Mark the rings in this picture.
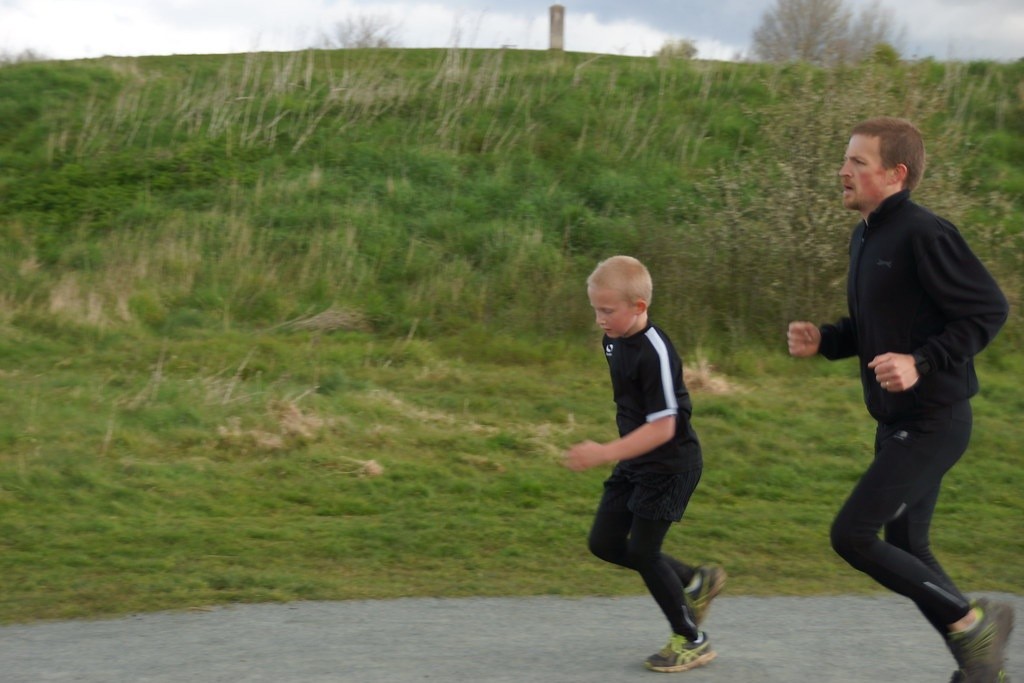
[886,381,888,385]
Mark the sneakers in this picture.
[684,560,727,626]
[644,631,717,673]
[942,598,1014,682]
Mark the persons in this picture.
[788,118,1016,682]
[558,255,727,672]
